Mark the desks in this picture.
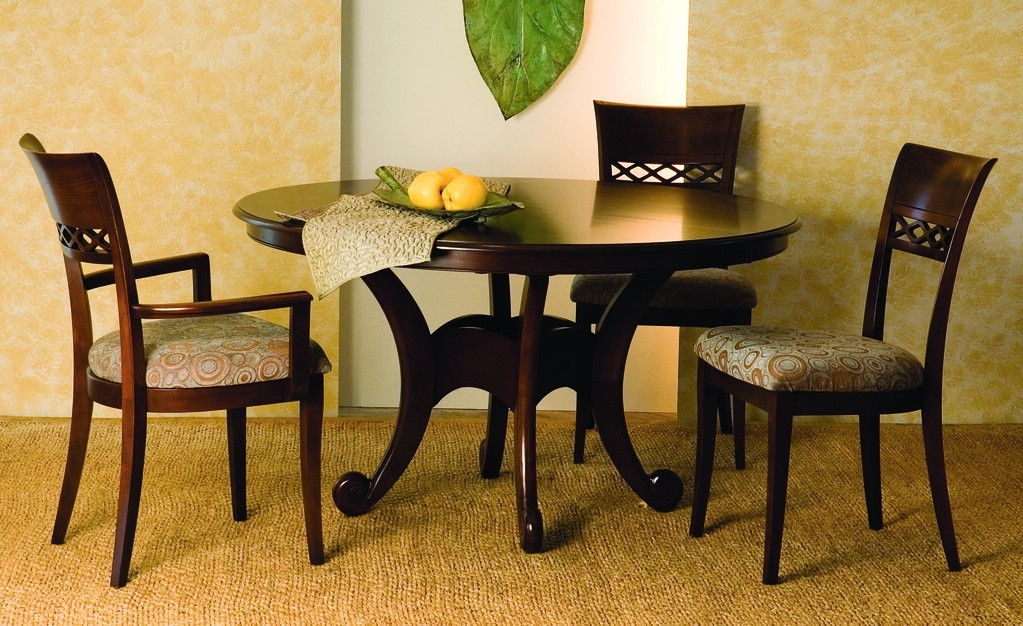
[229,177,806,558]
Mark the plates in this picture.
[373,165,525,217]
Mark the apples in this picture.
[408,167,488,210]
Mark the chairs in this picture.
[571,100,755,475]
[688,139,1002,585]
[19,129,338,594]
